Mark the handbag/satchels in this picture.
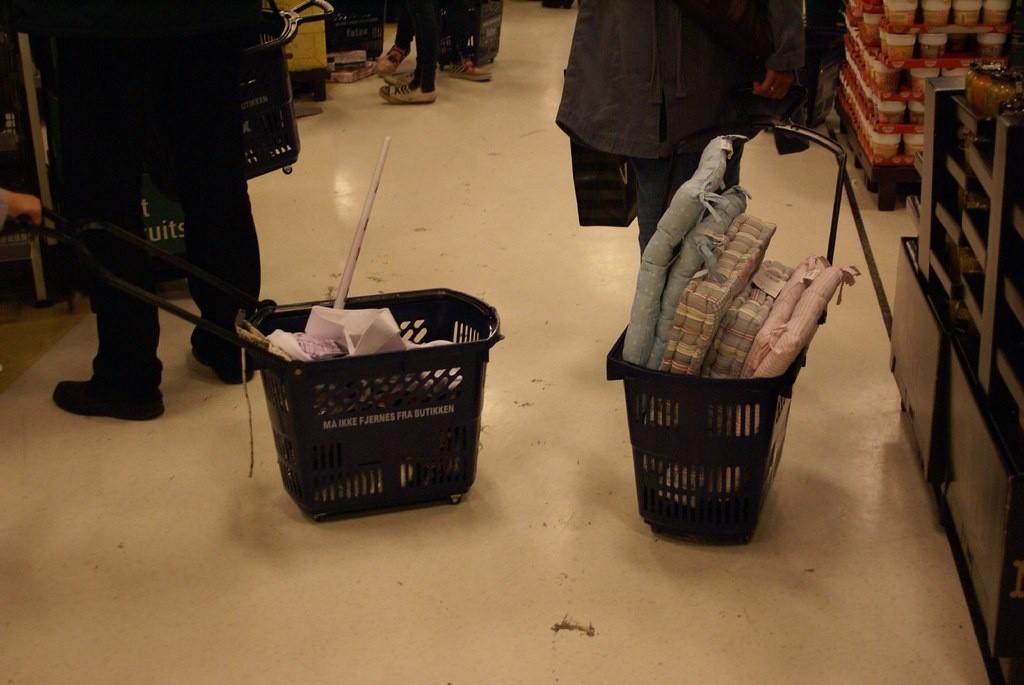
[569,136,636,227]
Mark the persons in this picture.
[0,189,44,231]
[2,1,262,423]
[555,0,814,285]
[373,0,492,104]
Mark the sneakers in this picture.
[448,60,492,81]
[379,85,436,104]
[385,74,414,86]
[376,44,411,75]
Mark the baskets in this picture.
[606,120,847,546]
[27,202,502,521]
[241,1,333,181]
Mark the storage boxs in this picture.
[327,50,377,83]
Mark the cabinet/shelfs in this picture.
[888,74,1024,685]
[0,31,59,301]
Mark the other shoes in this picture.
[52,379,165,421]
[193,346,253,383]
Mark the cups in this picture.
[838,0,1024,162]
